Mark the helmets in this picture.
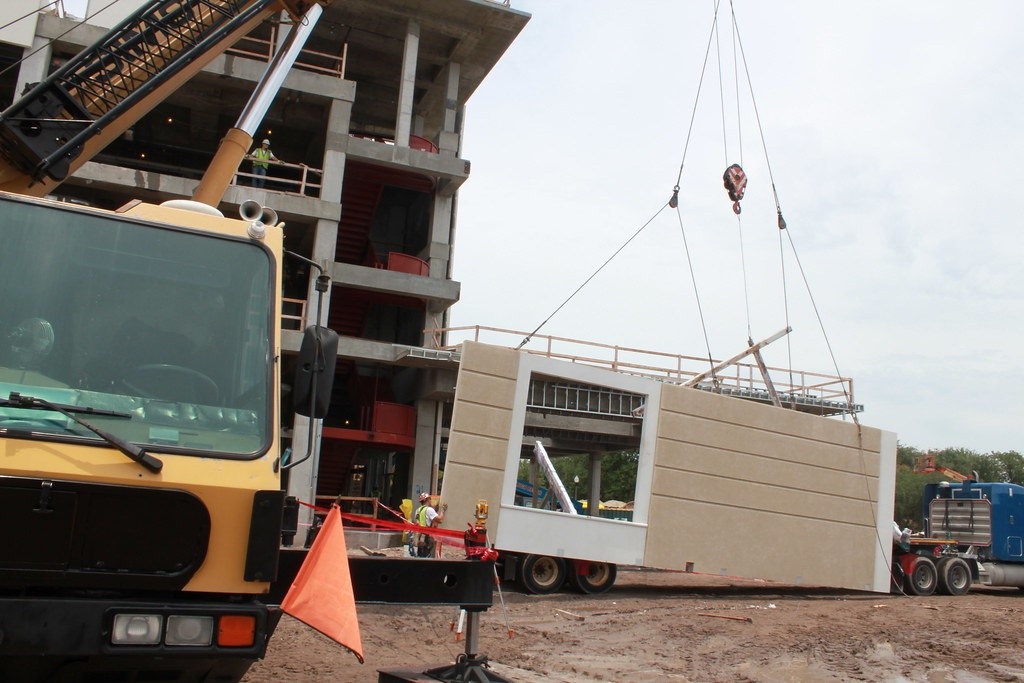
[418,492,431,501]
[263,139,270,145]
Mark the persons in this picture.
[415,493,448,557]
[248,139,284,188]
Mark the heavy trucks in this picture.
[493,471,1024,598]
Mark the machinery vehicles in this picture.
[0,0,514,683]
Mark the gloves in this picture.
[278,160,284,164]
[441,503,448,513]
